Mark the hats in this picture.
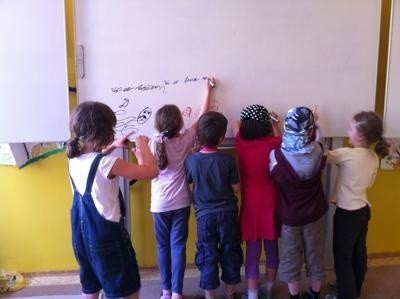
[240,103,270,125]
[283,106,315,136]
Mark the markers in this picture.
[270,114,280,123]
[208,79,215,88]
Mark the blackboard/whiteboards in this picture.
[73,0,382,143]
[0,0,71,144]
[381,0,400,138]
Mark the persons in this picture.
[233,104,281,299]
[268,104,329,299]
[147,78,215,299]
[65,101,158,299]
[324,110,389,299]
[185,111,244,299]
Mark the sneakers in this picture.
[241,278,272,298]
[302,286,320,299]
[325,279,354,299]
